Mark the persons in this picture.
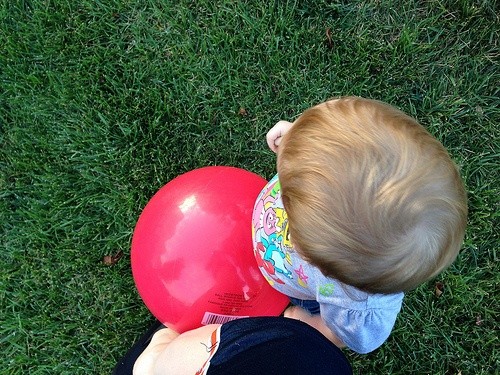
[116,310,354,375]
[250,93,469,355]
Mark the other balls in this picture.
[130,167,290,334]
